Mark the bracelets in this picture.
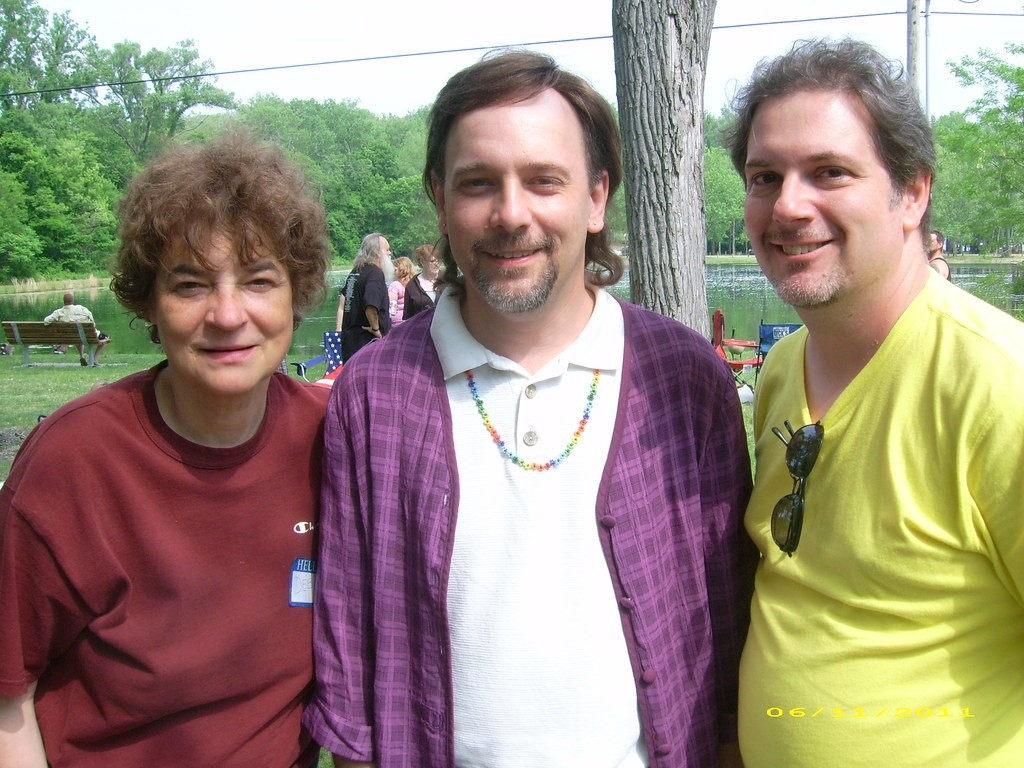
[371,327,379,332]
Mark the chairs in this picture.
[710,308,804,389]
[292,331,343,384]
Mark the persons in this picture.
[0,120,336,768]
[402,243,446,321]
[337,233,395,366]
[44,294,109,366]
[302,50,760,768]
[386,256,416,327]
[927,231,952,283]
[724,36,1024,768]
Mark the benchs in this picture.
[1,321,112,367]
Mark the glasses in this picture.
[771,419,825,556]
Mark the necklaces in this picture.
[464,365,600,470]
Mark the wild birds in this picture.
[0,342,16,354]
[52,344,69,355]
